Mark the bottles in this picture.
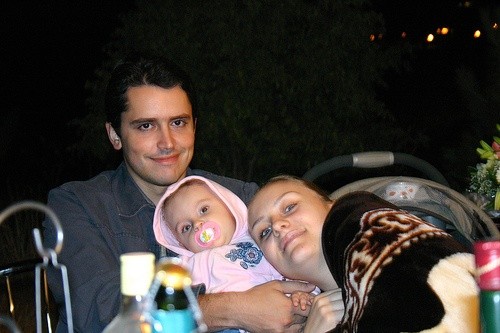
[149,263,198,333]
[474,241,500,333]
[102,252,155,333]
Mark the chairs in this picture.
[300,151,500,254]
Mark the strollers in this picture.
[302,151,499,245]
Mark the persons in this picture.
[153,176,322,333]
[44,56,316,332]
[248,176,481,333]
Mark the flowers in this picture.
[467,124,500,212]
